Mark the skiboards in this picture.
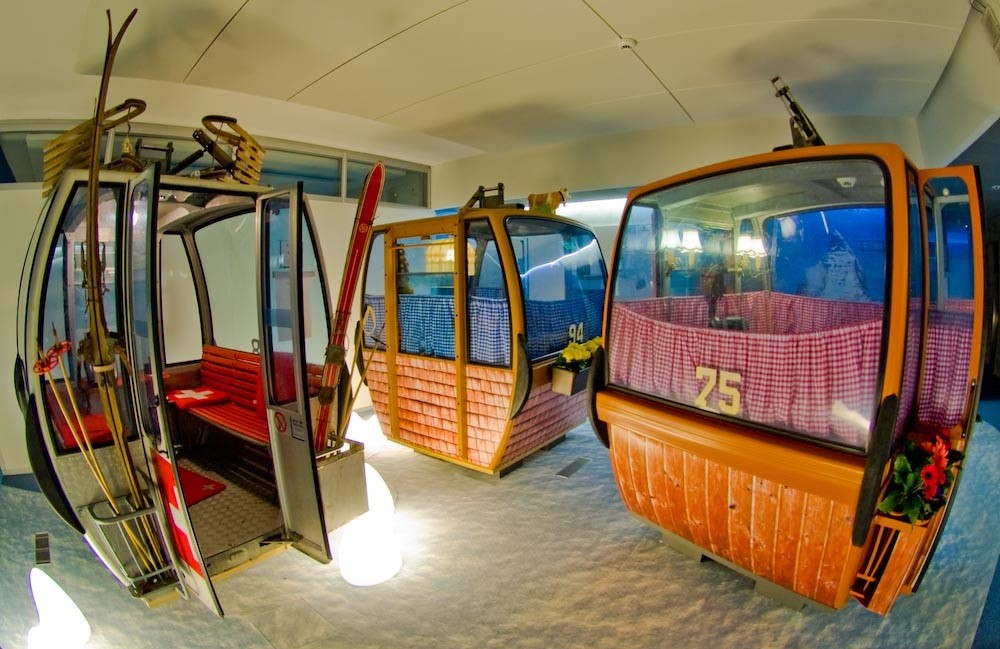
[85,6,180,580]
[311,159,387,461]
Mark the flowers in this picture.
[876,435,966,520]
[556,336,601,370]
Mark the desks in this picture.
[104,373,169,391]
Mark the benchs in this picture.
[172,345,325,447]
[46,393,132,452]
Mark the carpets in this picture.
[178,466,226,507]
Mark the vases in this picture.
[550,365,591,396]
[853,491,949,616]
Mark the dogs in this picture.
[527,186,571,215]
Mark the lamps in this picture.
[727,236,770,277]
[662,228,704,277]
